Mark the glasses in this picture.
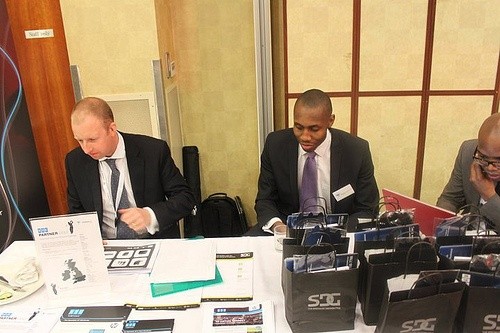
[473,145,500,169]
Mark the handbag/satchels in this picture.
[281,195,500,333]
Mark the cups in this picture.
[274,226,288,252]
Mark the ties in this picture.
[104,159,135,239]
[301,151,318,212]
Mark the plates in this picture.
[0,257,46,306]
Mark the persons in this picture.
[436,111,500,238]
[242,89,379,237]
[65,97,195,245]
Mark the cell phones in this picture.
[479,165,487,176]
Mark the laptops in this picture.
[382,188,458,237]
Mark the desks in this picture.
[0,229,375,333]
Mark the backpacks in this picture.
[201,193,240,238]
